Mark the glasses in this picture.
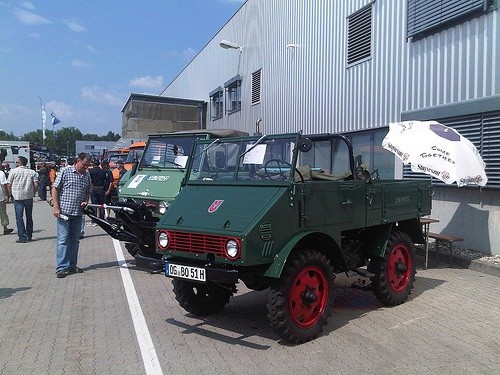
[80,160,90,166]
[16,162,20,164]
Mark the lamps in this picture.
[219,39,242,52]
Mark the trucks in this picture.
[81,118,263,259]
[59,139,168,201]
[154,129,432,344]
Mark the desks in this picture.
[417,218,440,269]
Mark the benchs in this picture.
[423,231,464,268]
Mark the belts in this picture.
[92,185,104,188]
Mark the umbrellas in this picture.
[381,119,488,269]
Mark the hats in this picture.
[171,144,179,150]
[115,160,124,165]
[101,160,109,164]
[91,159,100,164]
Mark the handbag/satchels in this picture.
[47,198,53,206]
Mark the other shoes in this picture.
[65,267,83,273]
[57,271,65,278]
[85,222,98,226]
[4,229,14,234]
[16,240,26,243]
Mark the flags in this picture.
[50,112,61,125]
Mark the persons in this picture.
[51,152,91,277]
[0,159,14,235]
[6,156,39,243]
[2,155,127,227]
